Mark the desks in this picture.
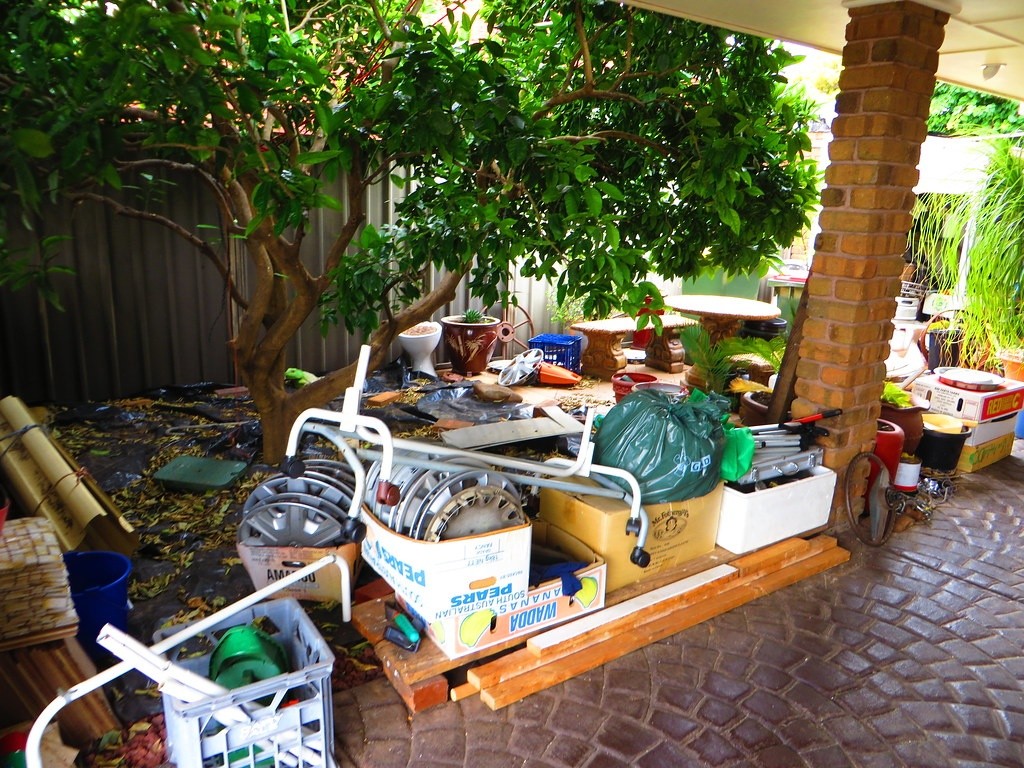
[664,295,781,397]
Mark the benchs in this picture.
[724,351,783,427]
[570,315,698,382]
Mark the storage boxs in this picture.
[612,373,658,405]
[60,551,131,657]
[631,380,689,402]
[921,291,954,319]
[963,411,1019,446]
[396,517,607,660]
[359,501,531,618]
[928,330,962,373]
[528,333,582,375]
[235,540,359,603]
[915,421,973,470]
[715,465,838,556]
[956,431,1015,473]
[863,419,905,513]
[533,477,723,593]
[912,369,1024,424]
[1014,408,1024,440]
[149,597,337,768]
[892,295,918,320]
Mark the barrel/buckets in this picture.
[62,552,132,661]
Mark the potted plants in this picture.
[441,309,501,377]
[880,380,930,455]
[995,338,1024,382]
[739,388,772,426]
[893,452,921,492]
[397,319,443,377]
